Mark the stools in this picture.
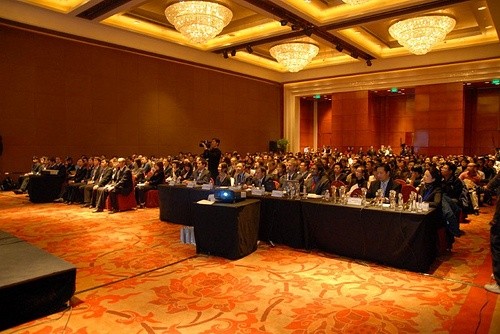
[67,182,161,210]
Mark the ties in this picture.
[382,184,386,197]
[288,175,292,180]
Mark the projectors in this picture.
[214,188,246,199]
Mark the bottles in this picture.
[325,190,330,201]
[251,184,255,194]
[236,183,242,192]
[209,180,212,189]
[177,178,180,184]
[260,185,265,195]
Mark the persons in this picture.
[202,138,222,172]
[482,170,500,293]
[14,143,500,251]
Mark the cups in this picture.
[423,203,429,211]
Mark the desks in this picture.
[157,182,442,275]
[193,199,260,260]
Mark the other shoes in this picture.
[109,209,118,214]
[84,203,96,209]
[475,210,479,215]
[92,208,103,213]
[14,189,23,194]
[484,283,500,294]
[64,200,73,205]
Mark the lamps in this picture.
[388,13,456,55]
[268,42,320,72]
[163,0,234,46]
